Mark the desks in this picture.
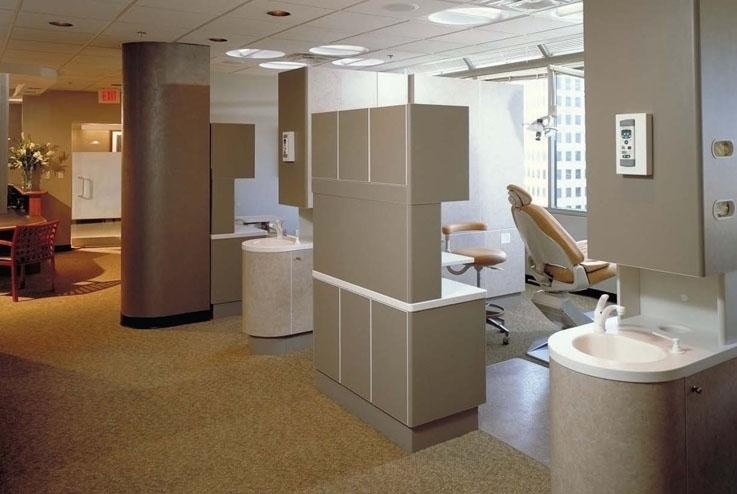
[0,213,47,274]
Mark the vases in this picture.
[19,168,33,189]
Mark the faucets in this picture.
[268,219,283,240]
[594,293,626,333]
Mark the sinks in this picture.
[573,331,668,364]
[250,237,293,248]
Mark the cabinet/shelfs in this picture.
[682,359,737,493]
[210,122,284,305]
[310,106,489,454]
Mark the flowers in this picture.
[6,132,57,168]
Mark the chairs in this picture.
[0,220,59,301]
[507,183,617,363]
[442,221,511,345]
